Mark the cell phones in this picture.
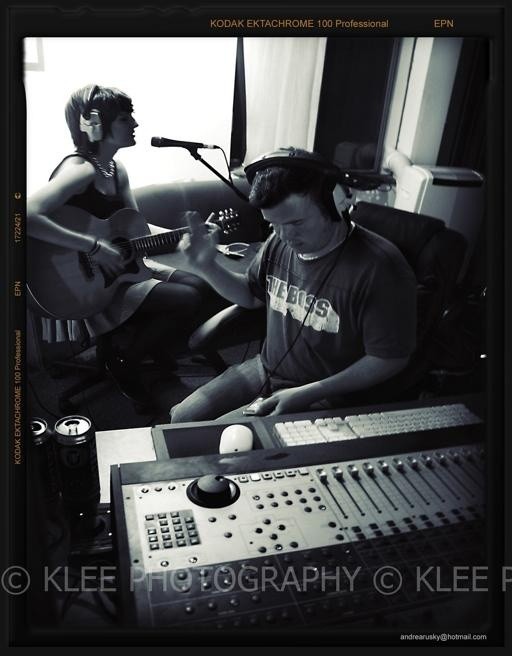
[242,393,272,415]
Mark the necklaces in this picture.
[91,153,116,178]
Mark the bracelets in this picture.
[84,236,103,256]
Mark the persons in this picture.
[27,87,205,398]
[171,153,421,428]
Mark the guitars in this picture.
[19,204,241,321]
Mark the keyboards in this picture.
[271,401,487,450]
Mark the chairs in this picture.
[350,199,450,406]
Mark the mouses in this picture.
[215,423,253,454]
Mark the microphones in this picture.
[149,134,216,159]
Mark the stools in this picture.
[27,286,164,415]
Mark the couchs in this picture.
[130,173,267,345]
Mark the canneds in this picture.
[53,415,100,508]
[28,417,58,502]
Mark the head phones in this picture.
[74,84,106,165]
[240,148,360,221]
